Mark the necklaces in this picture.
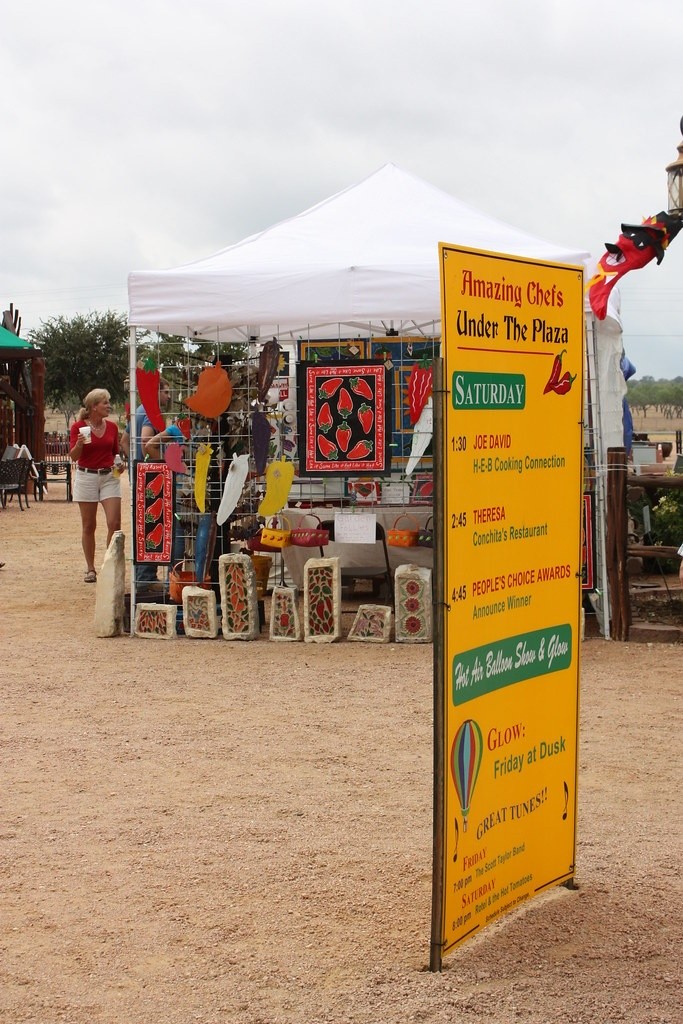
[89,420,105,430]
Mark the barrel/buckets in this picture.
[249,555,272,596]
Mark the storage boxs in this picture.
[122,592,169,633]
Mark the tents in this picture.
[126,163,628,638]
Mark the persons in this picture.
[70,389,125,583]
[120,377,171,592]
[145,421,200,573]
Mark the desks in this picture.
[36,462,74,501]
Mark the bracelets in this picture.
[76,443,83,447]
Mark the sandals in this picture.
[84,570,97,582]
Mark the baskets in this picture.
[290,514,329,547]
[387,514,419,547]
[169,561,211,603]
[418,515,433,547]
[260,515,291,548]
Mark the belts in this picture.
[79,467,114,475]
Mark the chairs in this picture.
[316,519,393,614]
[0,458,26,511]
[6,458,34,508]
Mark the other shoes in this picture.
[0,562,5,568]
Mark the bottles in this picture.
[113,453,122,478]
[350,483,357,506]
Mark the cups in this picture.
[79,426,91,444]
